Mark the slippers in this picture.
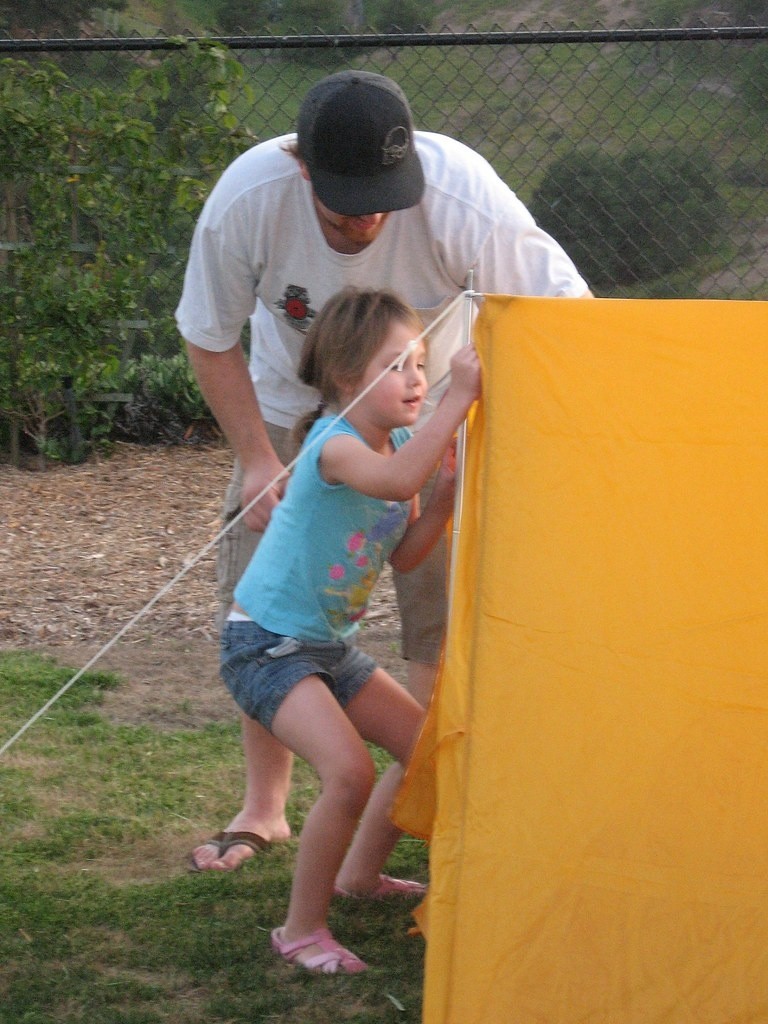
[271,927,369,975]
[185,831,271,874]
[333,874,426,901]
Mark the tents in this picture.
[386,299,768,1024]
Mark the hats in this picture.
[297,70,426,212]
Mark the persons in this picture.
[175,70,595,873]
[220,285,482,975]
